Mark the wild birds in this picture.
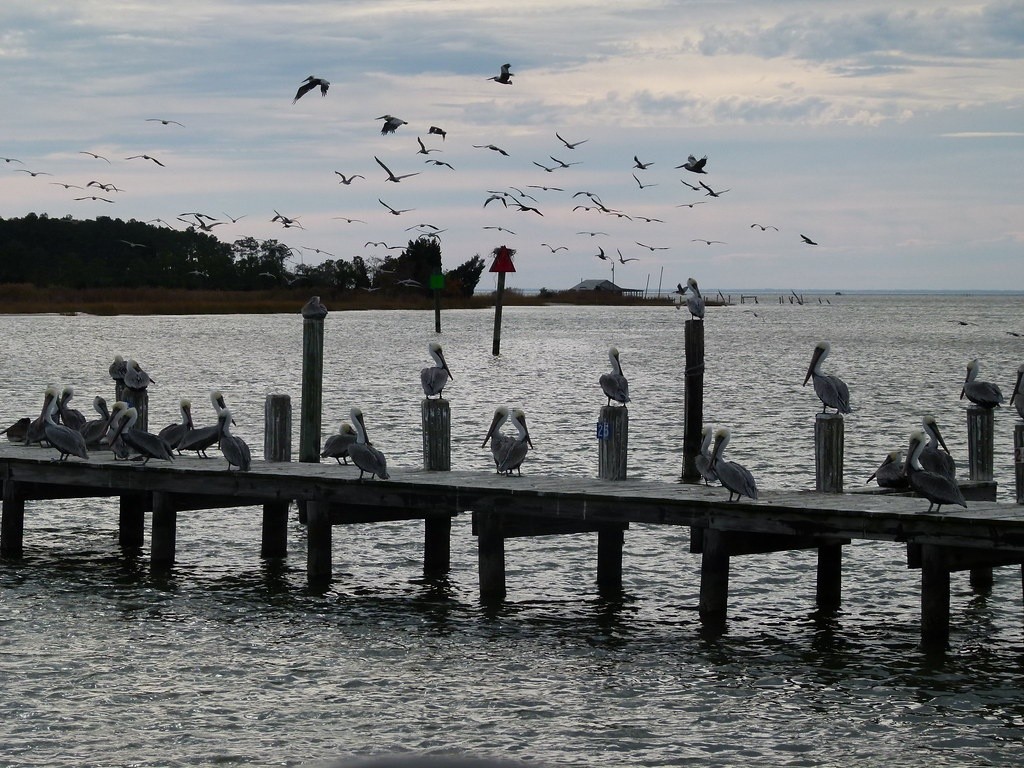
[686,277,705,319]
[348,406,390,482]
[1,357,236,466]
[918,414,956,480]
[673,283,689,295]
[901,430,968,513]
[706,428,758,503]
[294,62,821,275]
[318,424,358,465]
[867,450,912,488]
[420,342,454,400]
[599,347,632,407]
[949,319,977,327]
[481,406,534,478]
[803,340,853,415]
[1009,364,1024,420]
[0,118,306,279]
[960,358,1004,407]
[218,409,251,472]
[695,426,719,487]
